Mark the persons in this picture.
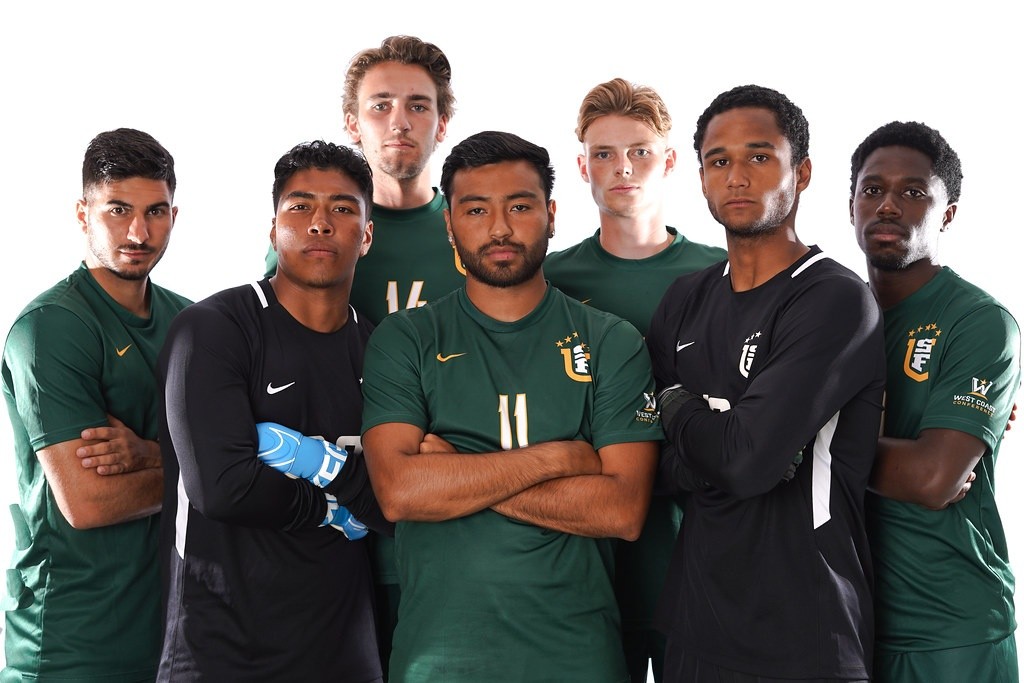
[156,139,383,683]
[645,85,888,683]
[0,127,195,683]
[360,131,668,683]
[542,78,728,683]
[263,36,466,683]
[848,122,1023,683]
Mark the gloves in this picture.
[781,444,807,482]
[651,368,683,406]
[256,422,348,488]
[318,492,369,540]
